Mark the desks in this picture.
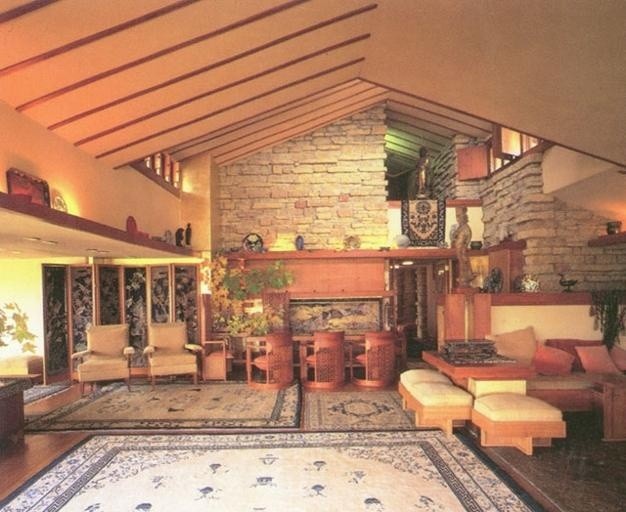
[420,349,537,393]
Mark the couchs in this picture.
[522,339,626,415]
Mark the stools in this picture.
[0,354,42,390]
[397,369,568,457]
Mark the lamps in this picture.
[386,143,432,201]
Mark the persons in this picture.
[416,147,429,194]
[451,205,473,288]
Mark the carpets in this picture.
[0,425,550,512]
[17,380,303,435]
[298,376,444,433]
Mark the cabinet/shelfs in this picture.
[0,381,27,454]
[589,378,626,445]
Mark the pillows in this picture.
[485,325,626,378]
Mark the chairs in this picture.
[201,322,400,391]
[143,322,203,390]
[70,322,135,398]
